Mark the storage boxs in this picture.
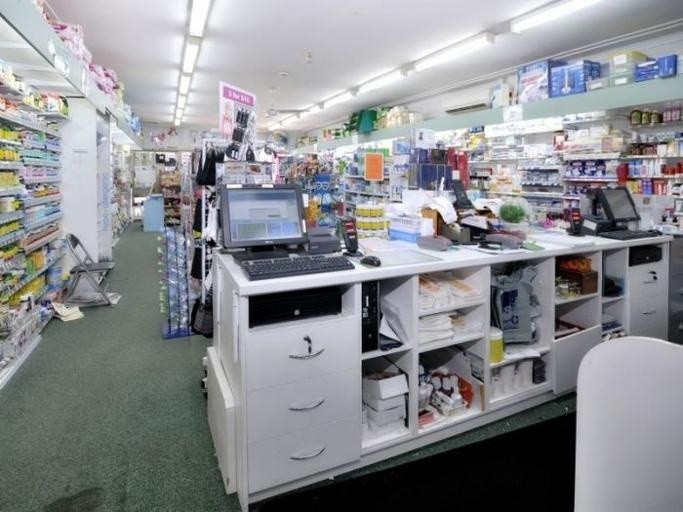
[585,51,683,92]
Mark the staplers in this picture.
[479,232,521,250]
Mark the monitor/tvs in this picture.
[222,183,309,262]
[598,186,642,231]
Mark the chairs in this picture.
[63,233,115,308]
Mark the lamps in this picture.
[267,65,409,133]
[413,32,495,72]
[509,0,600,33]
[174,0,214,127]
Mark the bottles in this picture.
[627,102,683,194]
[489,325,505,364]
[448,386,463,406]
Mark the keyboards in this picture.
[239,255,355,280]
[602,228,653,241]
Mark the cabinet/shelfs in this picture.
[207,235,675,512]
[1,76,68,388]
[344,98,683,237]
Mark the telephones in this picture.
[453,180,474,208]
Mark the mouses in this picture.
[360,255,382,267]
[648,228,662,235]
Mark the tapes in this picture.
[448,223,465,231]
